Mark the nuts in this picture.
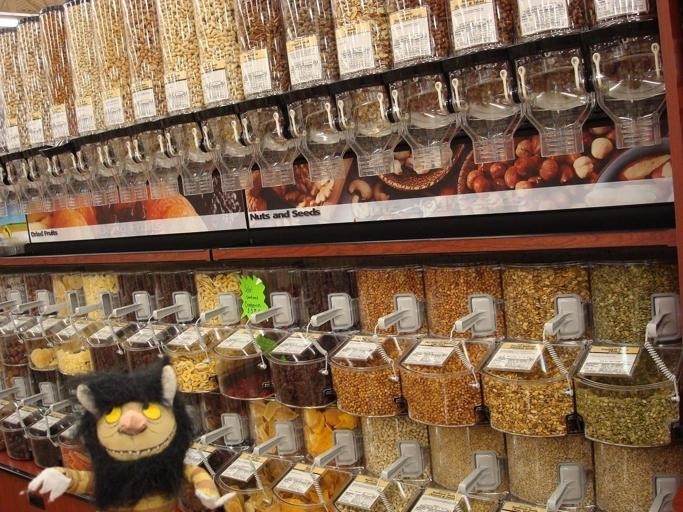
[30,126,679,511]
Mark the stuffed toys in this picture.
[28,353,237,512]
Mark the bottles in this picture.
[0,0,667,219]
